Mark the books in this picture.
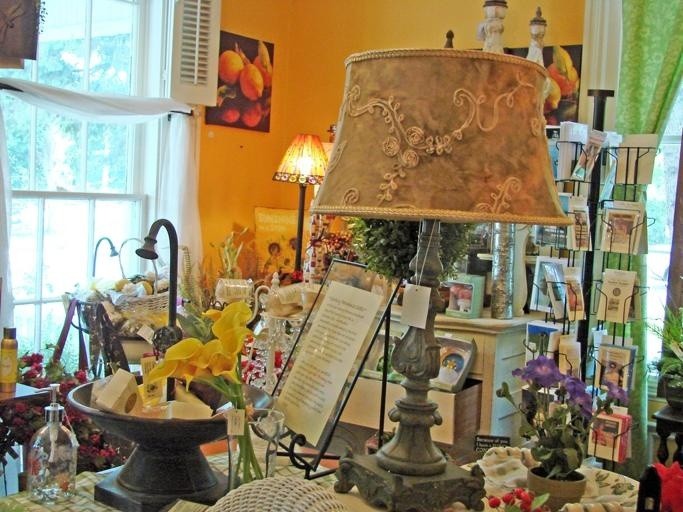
[525,321,561,367]
[593,414,631,461]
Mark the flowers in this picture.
[493,331,629,480]
[145,300,263,493]
[487,486,549,512]
[0,343,118,476]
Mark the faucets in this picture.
[136,217,179,325]
[91,238,117,278]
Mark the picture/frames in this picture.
[528,256,570,314]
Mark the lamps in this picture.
[311,47,574,512]
[272,134,327,281]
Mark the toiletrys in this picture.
[29,384,80,505]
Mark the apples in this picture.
[451,286,472,299]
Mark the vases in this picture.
[523,466,585,512]
[222,407,285,498]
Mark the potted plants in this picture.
[636,300,683,414]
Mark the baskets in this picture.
[102,237,169,314]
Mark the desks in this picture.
[0,382,509,512]
[338,304,550,445]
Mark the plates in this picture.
[575,465,639,512]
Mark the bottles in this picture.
[25,405,76,504]
[0,326,18,396]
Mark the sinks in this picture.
[68,375,273,493]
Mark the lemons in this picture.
[207,50,273,127]
[116,278,130,291]
[138,281,153,295]
[542,60,578,127]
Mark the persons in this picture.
[445,281,465,311]
[603,361,619,387]
[281,237,296,274]
[263,241,281,274]
[455,288,472,312]
[587,142,599,158]
